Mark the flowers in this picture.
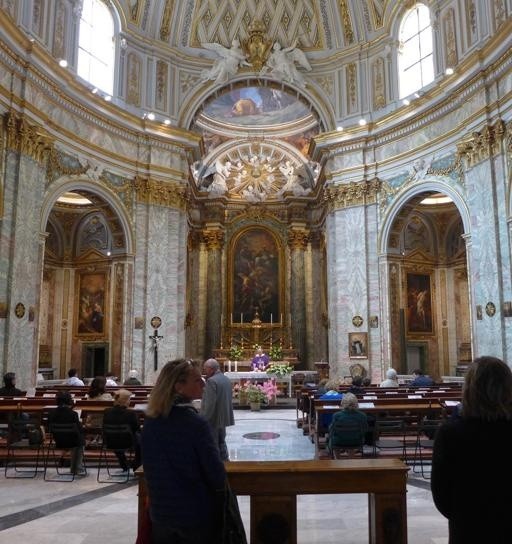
[232,383,280,401]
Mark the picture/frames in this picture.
[349,333,368,359]
[73,269,110,340]
[401,266,435,340]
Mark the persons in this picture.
[85,376,112,401]
[139,359,248,544]
[250,348,269,371]
[429,356,512,544]
[0,372,21,394]
[123,370,142,385]
[200,358,236,462]
[101,389,142,473]
[63,367,85,386]
[48,391,86,475]
[105,372,118,386]
[324,392,369,453]
[318,367,434,427]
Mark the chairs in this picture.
[329,419,440,479]
[7,417,142,484]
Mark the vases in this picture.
[249,401,261,412]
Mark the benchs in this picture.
[1,383,155,457]
[294,383,462,460]
